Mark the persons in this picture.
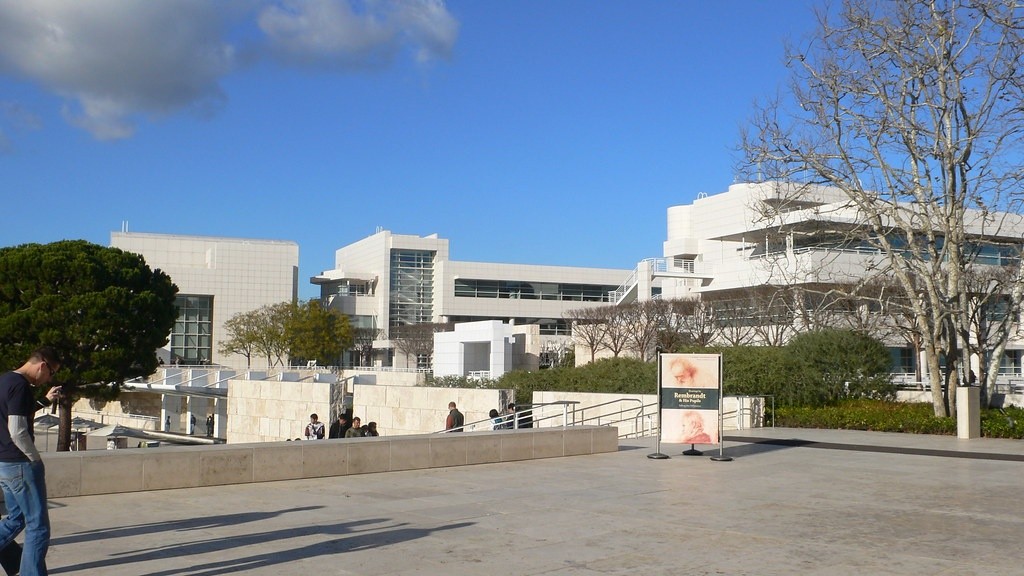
[443,402,466,432]
[164,415,214,438]
[285,415,380,441]
[670,358,698,388]
[489,404,522,430]
[136,442,162,447]
[0,348,65,575]
[159,357,164,364]
[677,411,712,444]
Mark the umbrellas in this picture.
[82,422,154,450]
[34,412,112,452]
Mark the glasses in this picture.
[42,358,55,376]
